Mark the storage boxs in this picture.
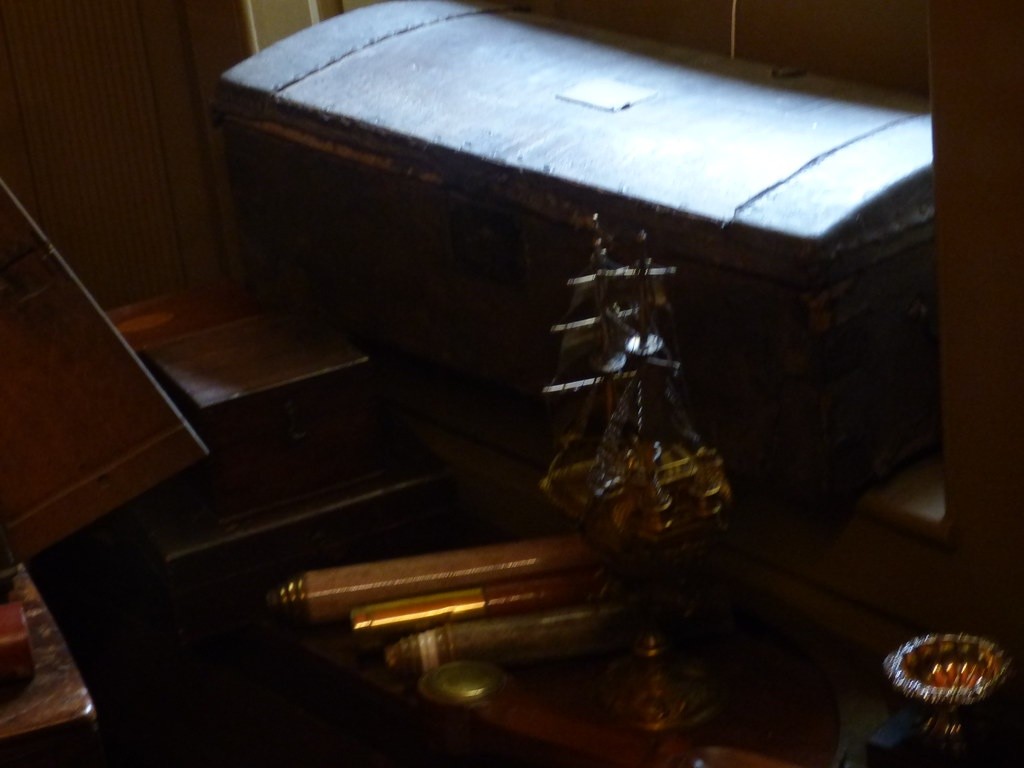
[121,0,934,653]
